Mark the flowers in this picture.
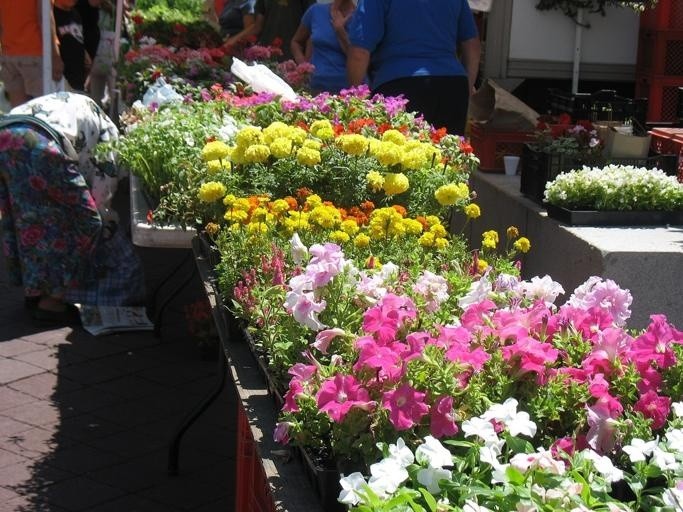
[196,187,682,512]
[96,0,483,226]
[516,110,682,225]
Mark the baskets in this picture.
[467,88,682,205]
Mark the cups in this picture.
[502,156,523,177]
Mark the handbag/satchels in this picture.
[64,220,146,311]
[89,30,116,79]
[469,78,540,133]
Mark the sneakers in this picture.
[25,296,81,320]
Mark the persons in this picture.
[53,2,133,120]
[198,1,482,138]
[0,89,120,328]
[0,0,64,108]
